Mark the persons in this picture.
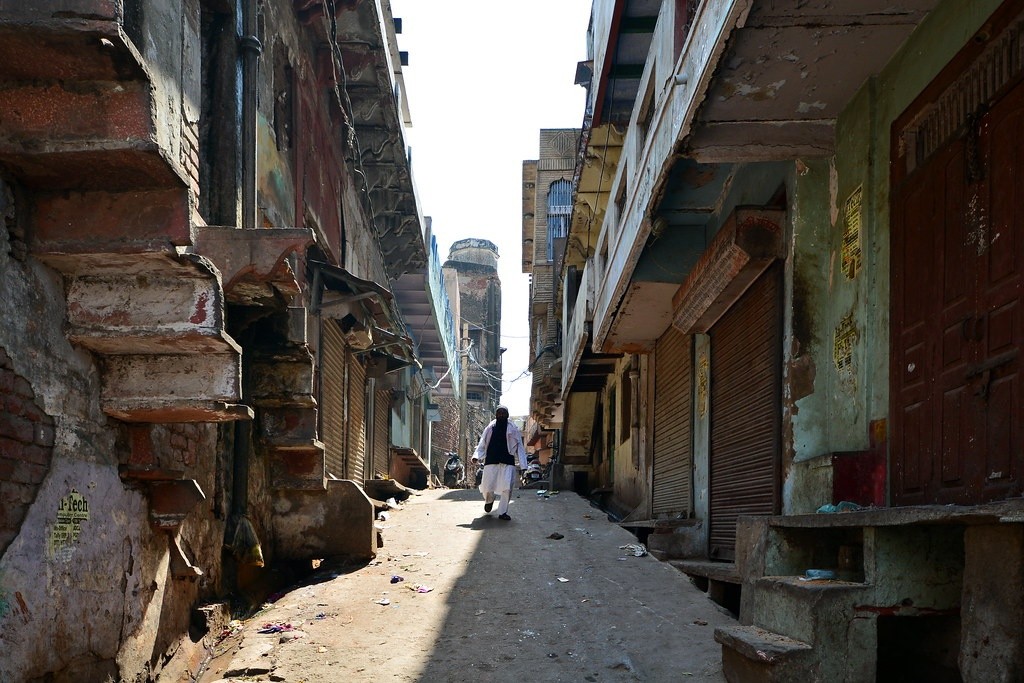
[471,405,527,520]
[444,454,461,488]
[474,463,484,488]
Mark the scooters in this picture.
[474,459,484,487]
[520,452,542,488]
[443,452,464,488]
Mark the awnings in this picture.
[309,259,422,373]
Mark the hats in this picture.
[496,405,508,412]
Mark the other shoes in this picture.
[499,513,511,520]
[485,502,493,512]
